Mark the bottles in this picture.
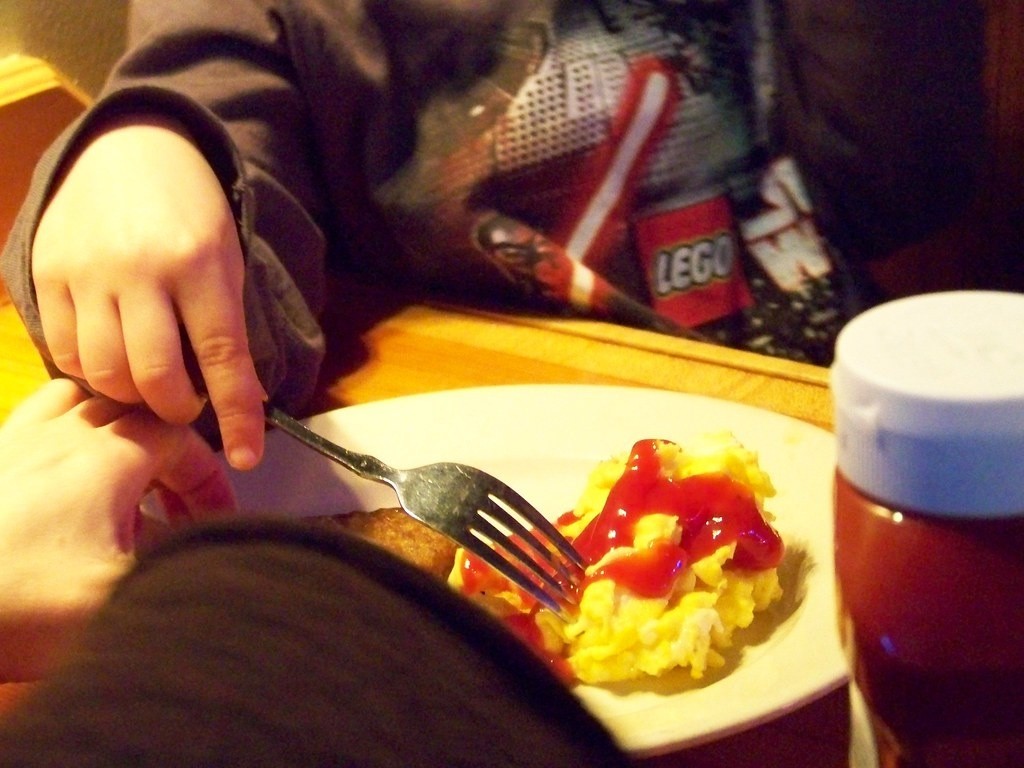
[830,289,1022,768]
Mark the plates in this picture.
[133,380,852,758]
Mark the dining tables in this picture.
[5,270,853,768]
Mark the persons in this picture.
[3,379,638,768]
[2,2,982,474]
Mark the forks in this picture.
[177,344,591,625]
